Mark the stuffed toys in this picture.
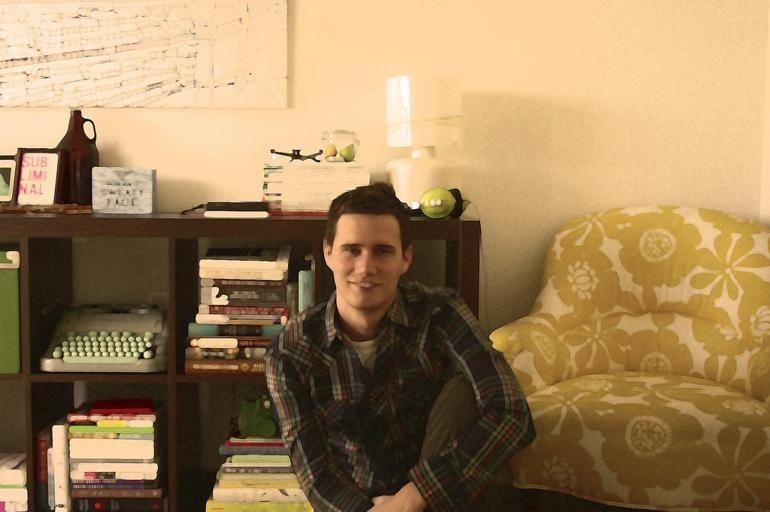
[237,394,281,438]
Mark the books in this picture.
[182,244,315,374]
[0,396,166,512]
[203,201,272,219]
[205,435,315,511]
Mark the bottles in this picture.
[386,146,455,205]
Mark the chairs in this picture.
[480,201,770,511]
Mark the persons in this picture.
[262,181,536,511]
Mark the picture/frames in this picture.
[9,146,65,208]
[0,153,20,208]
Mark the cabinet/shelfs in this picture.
[0,196,482,512]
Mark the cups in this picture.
[322,128,359,162]
